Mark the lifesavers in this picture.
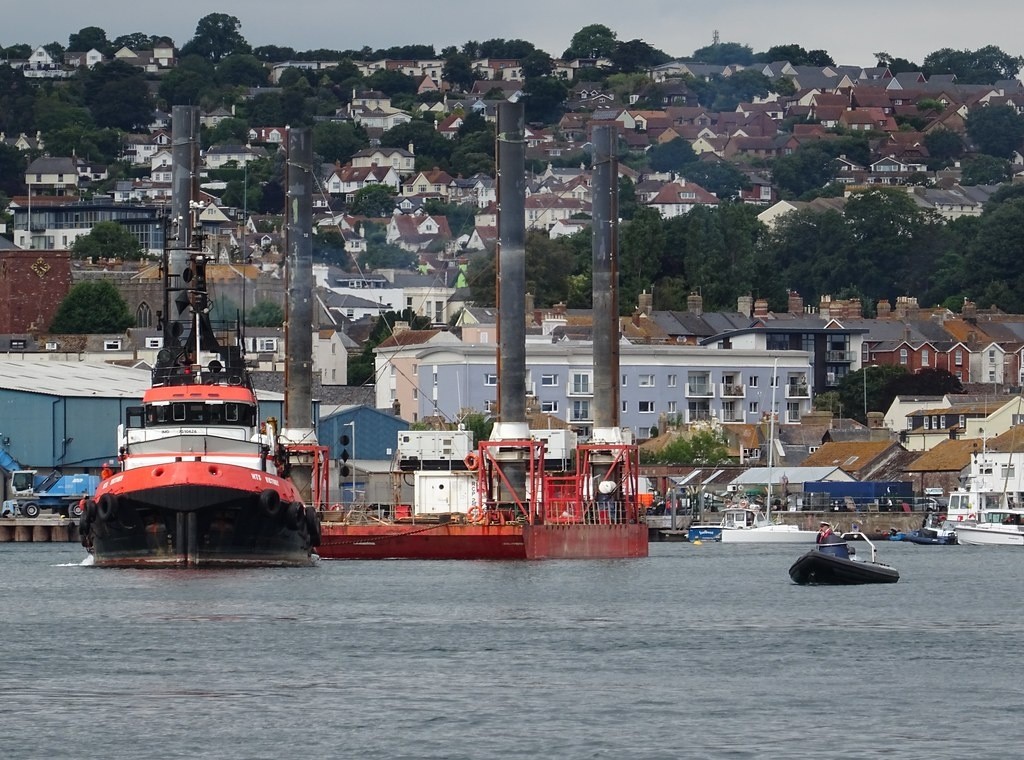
[469,506,485,522]
[464,453,480,469]
[330,505,344,511]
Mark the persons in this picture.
[816,522,835,544]
[101,463,113,480]
[676,498,679,515]
[666,499,671,516]
[887,498,893,512]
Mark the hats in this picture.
[819,521,830,527]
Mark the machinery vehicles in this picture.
[0,445,103,517]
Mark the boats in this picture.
[73,207,1024,563]
[788,530,899,584]
